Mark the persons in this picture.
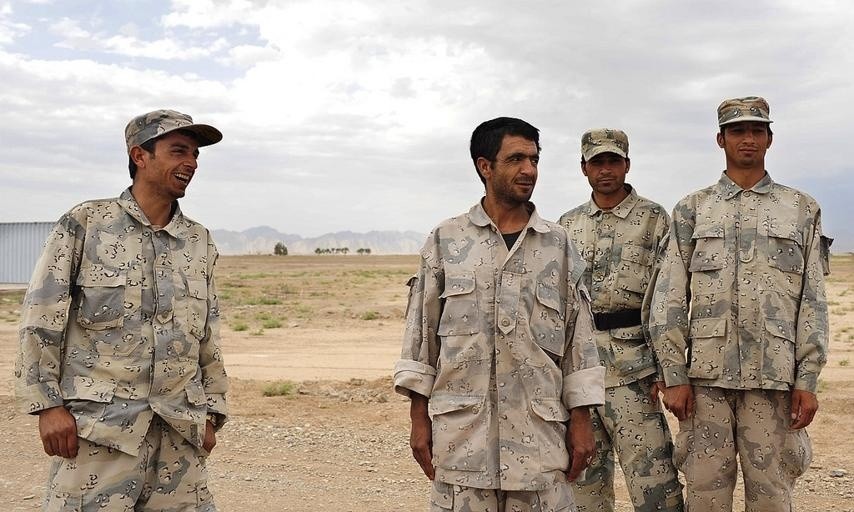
[393,118,607,512]
[669,96,834,511]
[13,109,231,512]
[554,129,685,512]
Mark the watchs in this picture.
[207,414,218,427]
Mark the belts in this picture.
[591,308,643,331]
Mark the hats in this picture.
[716,96,772,126]
[123,109,223,154]
[580,129,629,162]
